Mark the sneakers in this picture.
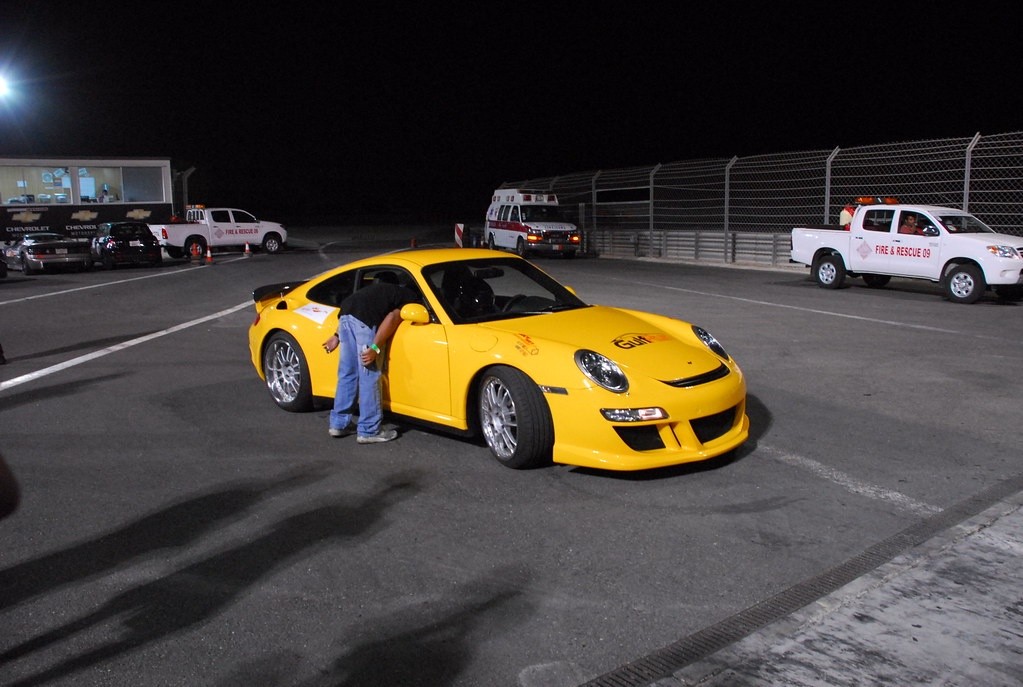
[357,427,398,443]
[329,419,358,436]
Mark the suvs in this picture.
[484,188,582,260]
[91,222,162,270]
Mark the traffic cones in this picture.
[203,247,221,268]
[243,241,257,260]
[190,242,203,260]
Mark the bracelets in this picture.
[371,345,381,354]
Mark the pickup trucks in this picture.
[146,205,288,258]
[788,204,1023,306]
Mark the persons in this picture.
[899,212,925,235]
[322,281,419,444]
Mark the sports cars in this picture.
[248,247,751,473]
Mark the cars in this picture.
[0,232,93,275]
